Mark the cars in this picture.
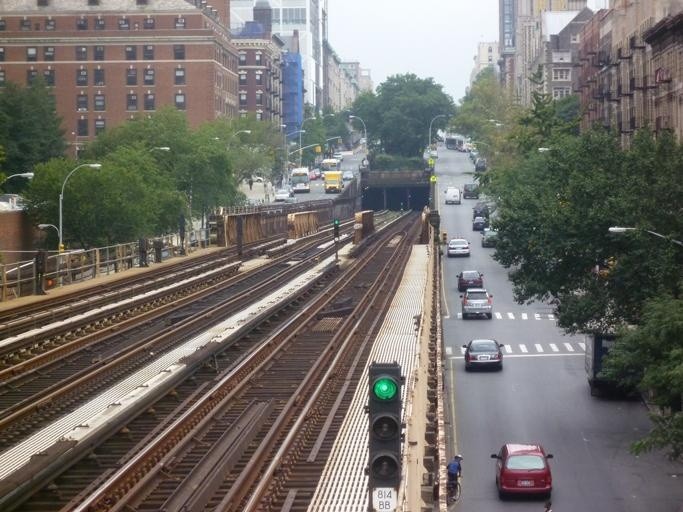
[439,126,505,372]
[274,152,353,203]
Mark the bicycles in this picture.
[446,473,461,505]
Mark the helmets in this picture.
[454,455,463,460]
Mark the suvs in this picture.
[490,442,554,500]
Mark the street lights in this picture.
[35,164,102,287]
[608,227,682,248]
[428,114,453,160]
[536,145,552,154]
[147,146,170,153]
[488,119,505,130]
[186,137,222,219]
[225,114,368,174]
[0,172,34,186]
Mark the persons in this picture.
[445,453,463,501]
[544,500,553,512]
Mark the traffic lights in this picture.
[58,244,64,254]
[367,361,405,491]
[333,218,338,238]
